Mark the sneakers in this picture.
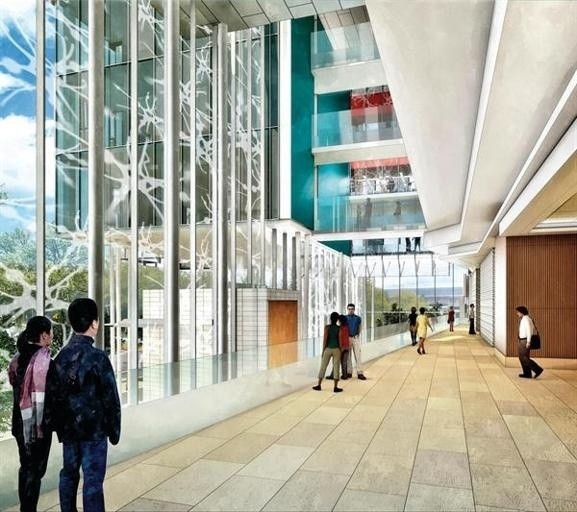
[534,368,544,378]
[311,373,366,393]
[518,371,533,379]
[417,349,426,355]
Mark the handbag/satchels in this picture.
[525,334,542,350]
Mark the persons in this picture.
[412,307,433,355]
[408,306,419,346]
[468,303,476,334]
[392,200,401,225]
[312,303,366,392]
[515,306,543,379]
[361,198,371,228]
[44,298,121,512]
[9,315,55,512]
[448,306,455,332]
